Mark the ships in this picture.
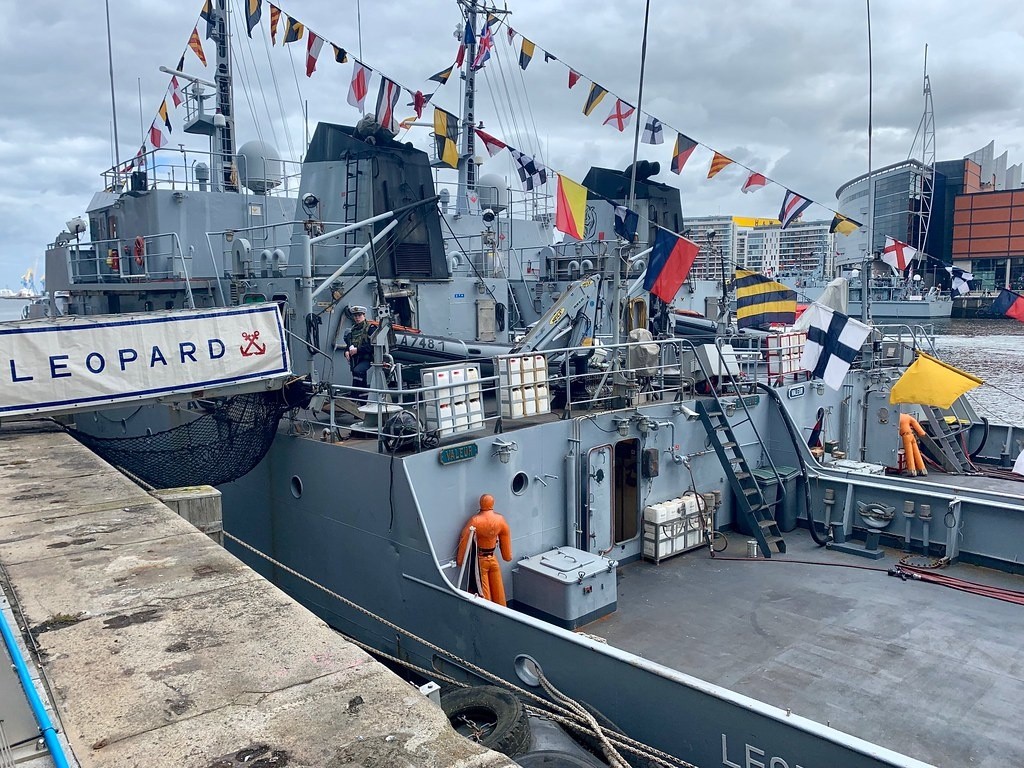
[24,0,1021,768]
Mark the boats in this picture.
[848,276,955,318]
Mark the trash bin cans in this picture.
[764,465,801,533]
[736,469,779,537]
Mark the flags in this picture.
[167,75,185,109]
[158,99,172,134]
[283,15,304,47]
[333,47,347,63]
[434,106,459,169]
[131,145,148,166]
[176,57,184,72]
[400,11,1024,410]
[149,120,168,148]
[121,165,132,172]
[188,27,207,67]
[270,3,281,47]
[245,0,262,39]
[375,75,401,132]
[199,0,216,40]
[306,30,324,77]
[347,59,373,113]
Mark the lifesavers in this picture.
[439,685,532,759]
[134,236,144,265]
[510,748,599,768]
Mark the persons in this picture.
[343,306,378,405]
[899,412,929,477]
[456,494,513,607]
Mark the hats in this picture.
[350,306,367,314]
